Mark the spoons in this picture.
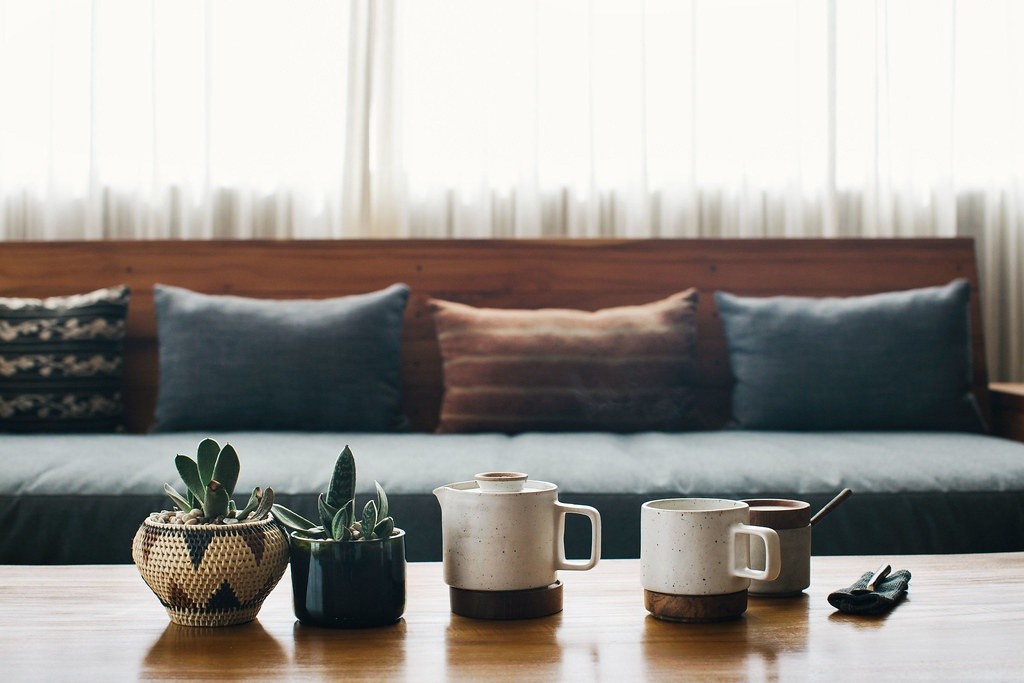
[850,564,891,596]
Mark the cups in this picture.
[640,498,781,622]
[738,499,811,598]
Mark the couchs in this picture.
[0,237,1024,557]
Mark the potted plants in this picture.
[271,445,408,627]
[132,438,290,628]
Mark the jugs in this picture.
[431,472,601,621]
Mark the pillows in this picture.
[713,276,982,432]
[0,282,142,435]
[148,280,408,433]
[424,288,700,433]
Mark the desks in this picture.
[0,551,1024,683]
